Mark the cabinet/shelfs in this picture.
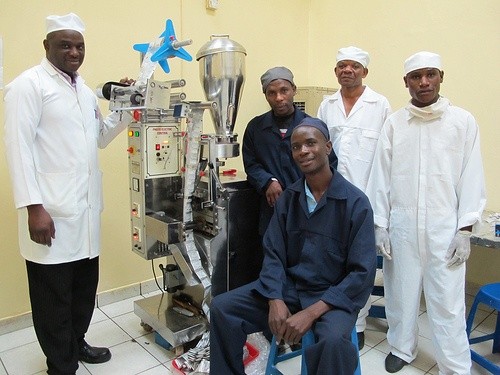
[293,87,339,117]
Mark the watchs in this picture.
[266,177,279,191]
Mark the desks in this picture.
[470,210,500,249]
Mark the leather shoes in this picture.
[79,343,111,364]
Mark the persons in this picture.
[369,51,485,375]
[4,13,138,375]
[221,45,393,375]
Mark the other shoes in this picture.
[357,331,364,351]
[385,353,405,373]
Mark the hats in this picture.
[293,116,329,141]
[403,50,441,75]
[335,46,370,68]
[260,67,294,93]
[46,12,85,38]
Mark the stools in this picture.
[466,284,500,375]
[265,326,363,375]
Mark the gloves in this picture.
[375,226,392,260]
[446,231,472,270]
